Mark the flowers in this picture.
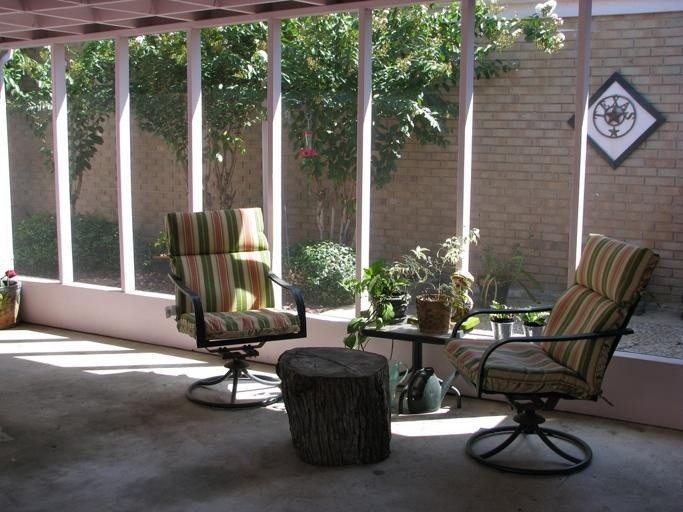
[0,269,16,286]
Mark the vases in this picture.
[0,283,23,330]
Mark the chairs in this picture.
[163,206,307,411]
[441,232,660,478]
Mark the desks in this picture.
[359,321,473,414]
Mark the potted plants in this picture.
[475,236,544,309]
[487,300,521,341]
[522,305,550,338]
[340,227,481,351]
